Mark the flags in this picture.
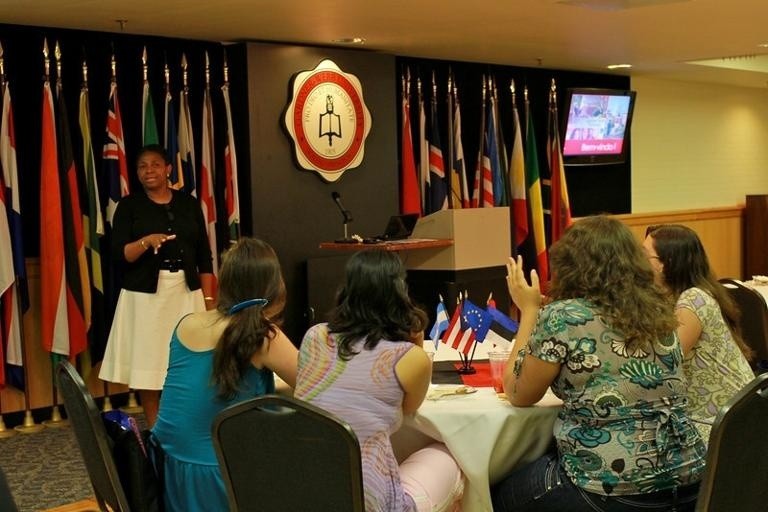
[428,304,451,352]
[2,77,244,392]
[461,304,493,344]
[442,305,478,357]
[401,92,572,298]
[485,306,520,344]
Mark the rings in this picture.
[505,276,512,280]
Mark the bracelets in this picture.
[141,238,149,253]
[204,297,216,302]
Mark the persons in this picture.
[148,235,300,511]
[104,143,220,437]
[572,99,624,139]
[489,213,708,512]
[292,248,466,512]
[640,223,764,460]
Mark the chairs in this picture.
[212,394,364,512]
[56,360,129,512]
[695,278,767,512]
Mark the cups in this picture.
[488,350,515,398]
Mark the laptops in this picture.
[373,214,418,239]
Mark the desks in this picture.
[391,383,565,512]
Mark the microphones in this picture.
[331,191,352,222]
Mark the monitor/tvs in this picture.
[562,87,637,165]
